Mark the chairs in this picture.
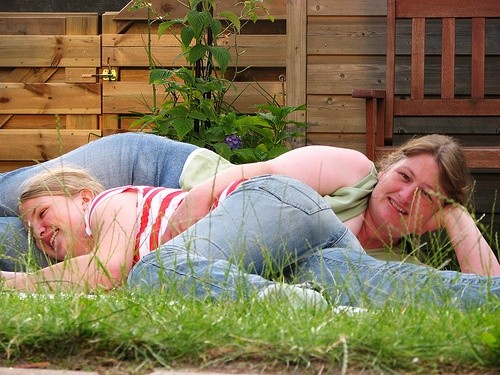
[352,1,500,173]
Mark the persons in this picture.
[0,130,499,272]
[0,164,500,322]
